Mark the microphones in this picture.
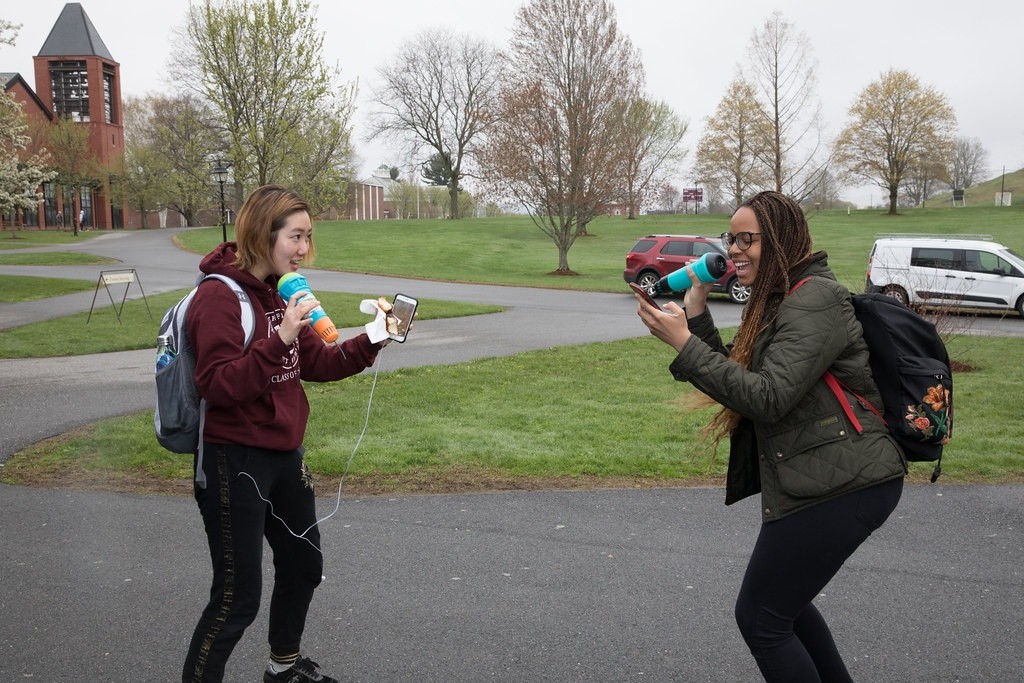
[277,272,346,359]
[654,252,727,296]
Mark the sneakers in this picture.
[264,655,338,683]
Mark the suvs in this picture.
[622,233,754,305]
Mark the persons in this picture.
[182,184,419,683]
[56,210,89,232]
[634,190,909,683]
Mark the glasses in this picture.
[721,232,761,251]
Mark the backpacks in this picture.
[154,271,255,454]
[788,274,953,481]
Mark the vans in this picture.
[864,233,1024,322]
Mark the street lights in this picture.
[210,160,229,242]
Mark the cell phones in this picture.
[387,293,419,343]
[629,282,662,311]
